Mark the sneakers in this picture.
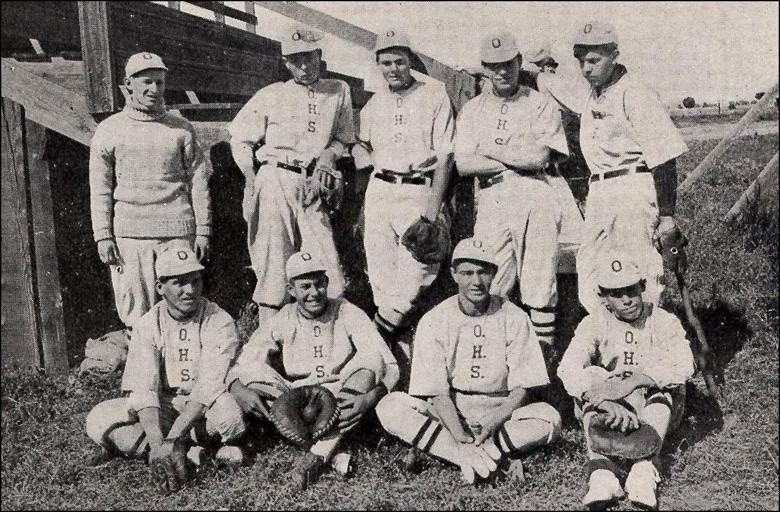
[579,468,628,509]
[186,446,206,467]
[281,450,330,491]
[329,446,355,478]
[625,459,663,509]
[217,446,243,469]
[397,438,440,473]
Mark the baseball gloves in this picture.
[587,411,663,462]
[268,383,342,449]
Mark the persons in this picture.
[88,53,214,350]
[84,248,244,493]
[376,239,560,484]
[558,260,695,509]
[538,56,558,74]
[464,22,689,315]
[352,28,456,337]
[227,25,353,327]
[227,252,398,487]
[458,29,569,364]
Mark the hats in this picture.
[155,246,207,281]
[450,236,500,266]
[124,50,168,80]
[597,254,645,290]
[478,30,524,66]
[371,27,412,58]
[284,249,329,282]
[278,27,323,59]
[570,19,621,51]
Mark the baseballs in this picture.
[302,404,319,423]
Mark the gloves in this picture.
[299,162,344,211]
[655,223,689,274]
[149,454,185,497]
[518,38,553,70]
[457,440,502,484]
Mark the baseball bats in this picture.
[676,269,720,397]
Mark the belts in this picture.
[367,171,431,185]
[255,158,315,176]
[583,162,647,183]
[473,167,546,189]
